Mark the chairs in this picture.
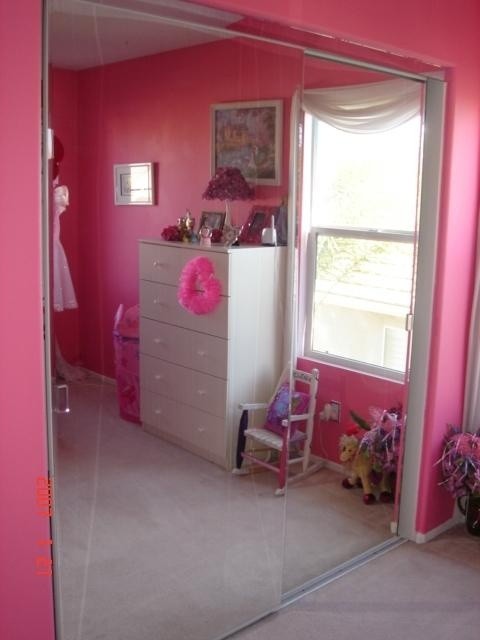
[232,363,324,498]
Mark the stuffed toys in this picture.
[339,430,393,504]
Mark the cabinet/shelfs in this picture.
[136,238,289,476]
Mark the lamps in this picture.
[202,166,256,243]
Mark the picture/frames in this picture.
[197,211,225,242]
[209,98,282,186]
[113,162,156,207]
